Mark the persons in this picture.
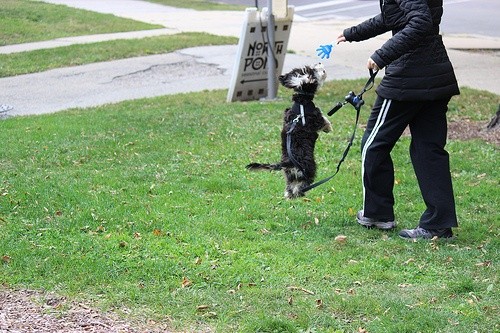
[336,0,461,241]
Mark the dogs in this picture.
[245,62,333,200]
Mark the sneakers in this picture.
[399,225,453,241]
[356,210,396,230]
